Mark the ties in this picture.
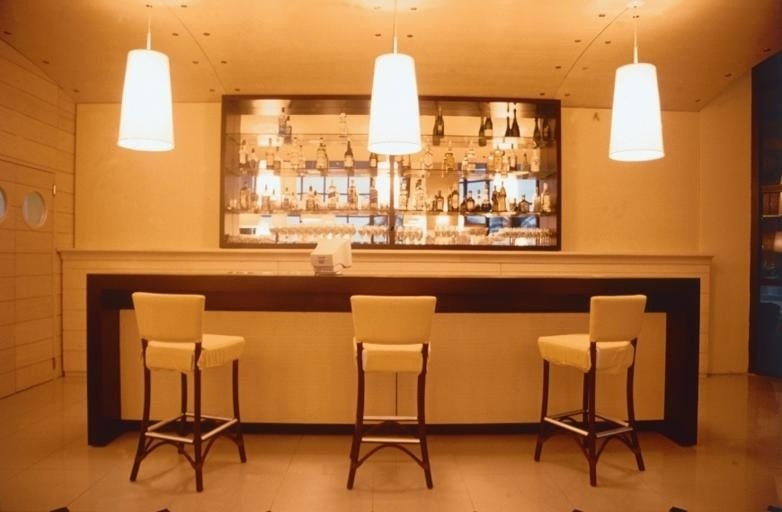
[310,236,352,274]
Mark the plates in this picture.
[219,93,565,251]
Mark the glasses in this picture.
[367,1,423,159]
[607,3,666,165]
[116,0,177,153]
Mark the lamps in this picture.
[344,293,438,490]
[128,291,247,492]
[533,290,645,487]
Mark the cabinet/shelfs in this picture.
[504,115,511,139]
[477,113,486,147]
[511,108,520,137]
[483,108,493,140]
[533,116,540,139]
[337,112,348,139]
[284,116,293,145]
[278,107,288,137]
[541,114,551,139]
[434,104,445,138]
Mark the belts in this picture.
[267,225,556,247]
